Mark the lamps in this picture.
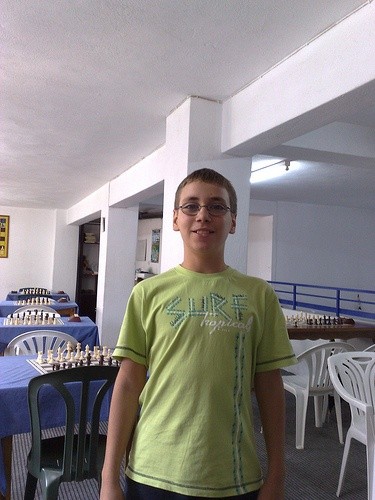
[250,158,293,183]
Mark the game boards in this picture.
[20,291,52,295]
[289,320,353,327]
[4,318,65,326]
[14,299,54,305]
[26,353,121,375]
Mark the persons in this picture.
[98,168,300,500]
[76,255,94,302]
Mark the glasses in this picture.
[176,201,235,216]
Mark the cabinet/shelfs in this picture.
[76,224,100,309]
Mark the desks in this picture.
[0,317,100,352]
[0,351,148,500]
[7,293,69,301]
[0,301,79,319]
[286,320,375,412]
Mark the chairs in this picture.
[23,366,119,500]
[15,306,61,317]
[261,340,375,500]
[3,330,81,357]
